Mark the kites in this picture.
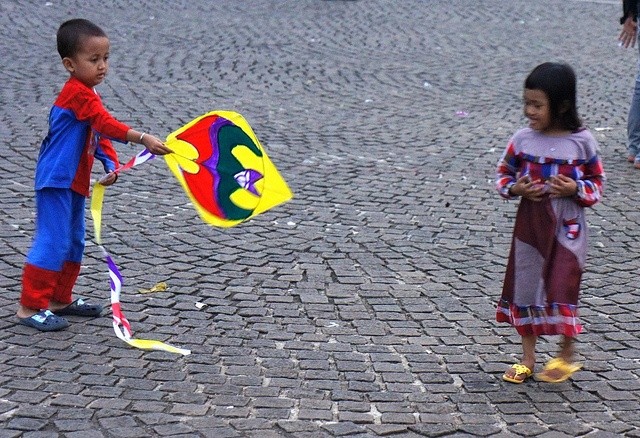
[92,112,293,357]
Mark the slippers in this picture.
[503,364,533,383]
[53,296,102,316]
[537,357,582,383]
[17,310,69,330]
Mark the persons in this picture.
[495,63,607,384]
[17,19,172,331]
[618,0,640,167]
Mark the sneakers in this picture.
[634,157,640,168]
[628,148,639,161]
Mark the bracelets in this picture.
[138,132,146,145]
